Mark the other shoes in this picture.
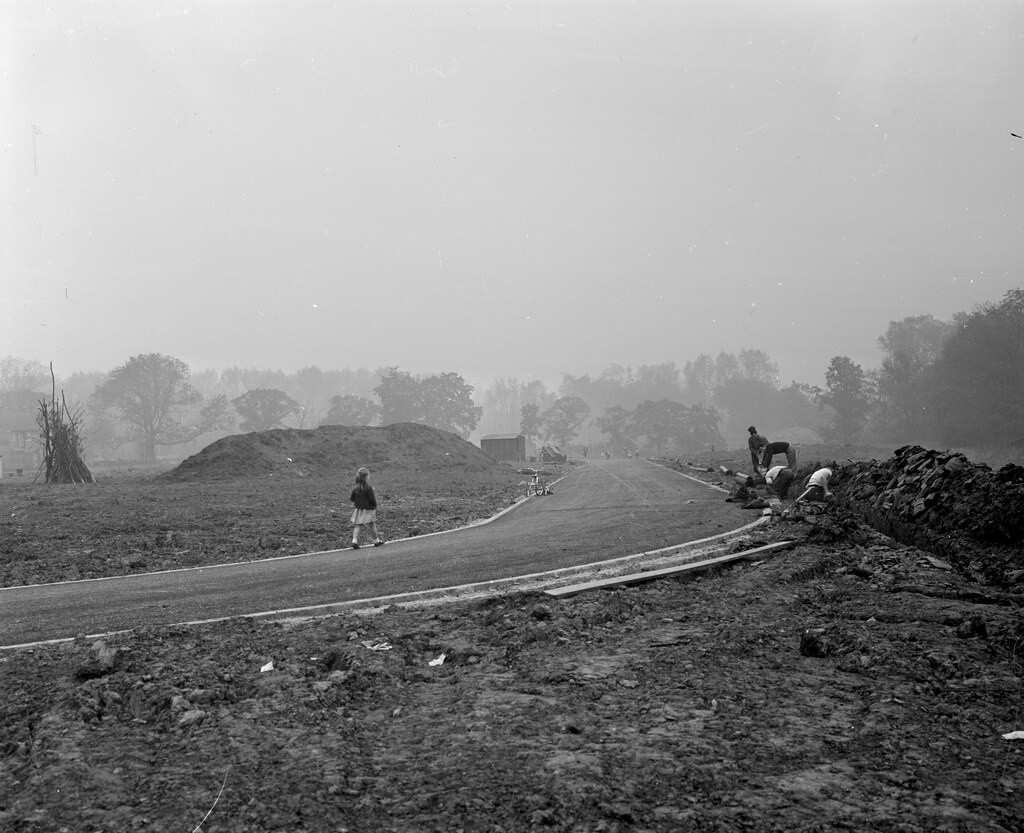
[375,540,385,547]
[351,542,359,549]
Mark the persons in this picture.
[758,441,798,473]
[760,465,795,500]
[349,468,385,548]
[804,463,836,502]
[747,426,770,473]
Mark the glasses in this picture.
[751,431,756,435]
